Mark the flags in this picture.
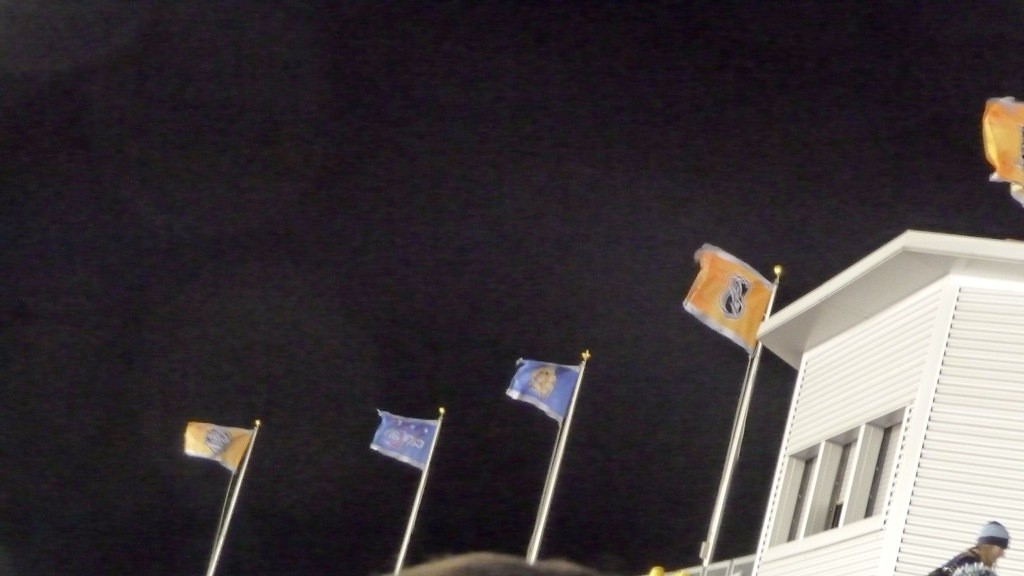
[506,359,580,423]
[184,422,253,473]
[982,96,1024,204]
[683,244,774,355]
[370,411,439,470]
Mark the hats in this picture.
[978,521,1008,548]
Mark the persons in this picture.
[928,521,1009,576]
